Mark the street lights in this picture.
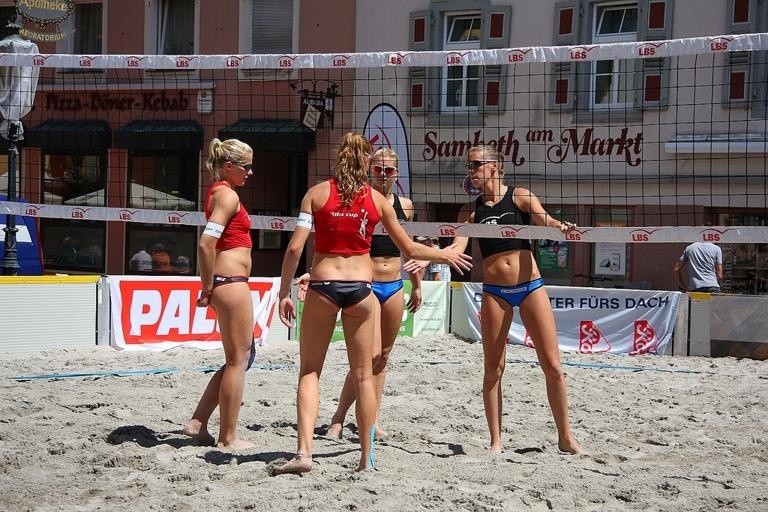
[1,34,41,281]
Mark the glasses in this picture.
[466,161,493,171]
[370,165,399,176]
[225,159,252,171]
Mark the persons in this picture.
[273,132,474,473]
[401,146,585,455]
[129,243,190,274]
[181,136,256,448]
[673,221,723,292]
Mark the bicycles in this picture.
[573,274,625,289]
